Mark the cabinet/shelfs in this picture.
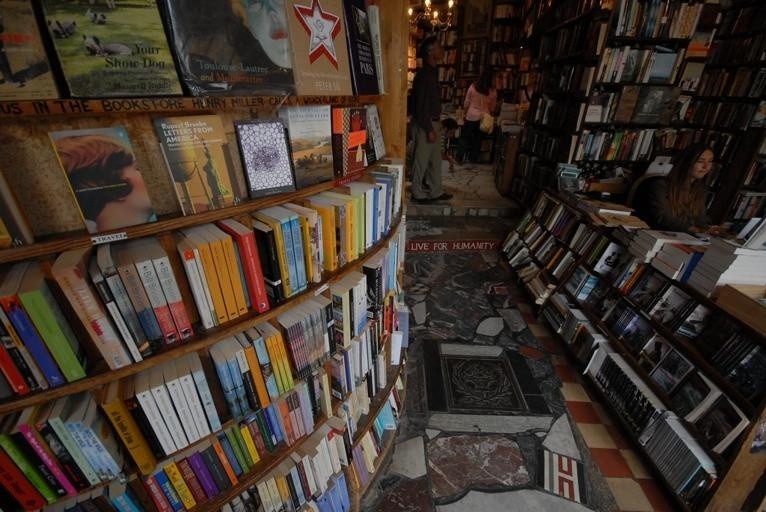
[1,0,416,510]
[482,194,766,511]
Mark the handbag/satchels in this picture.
[480,112,495,134]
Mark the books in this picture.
[499,2,765,512]
[408,1,499,210]
[1,1,412,512]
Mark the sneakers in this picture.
[411,195,433,204]
[433,194,453,201]
